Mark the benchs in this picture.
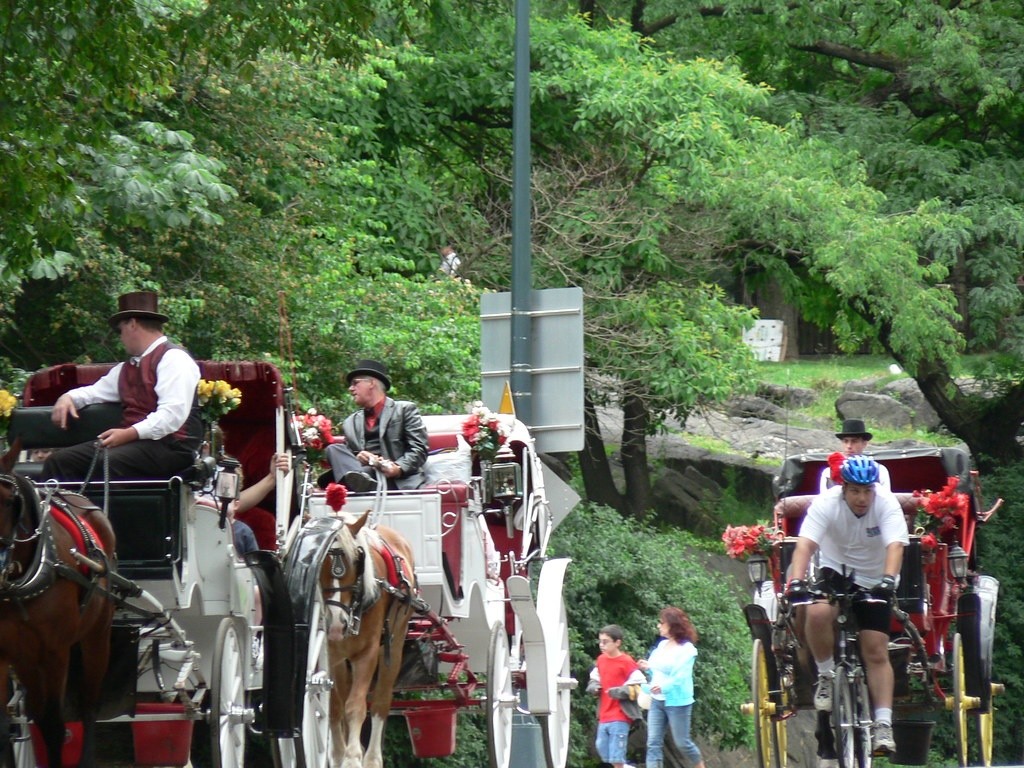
[10,360,972,697]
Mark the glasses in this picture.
[351,378,371,385]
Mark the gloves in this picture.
[871,575,895,602]
[787,579,808,602]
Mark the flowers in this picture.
[198,379,243,421]
[721,523,781,562]
[325,482,349,513]
[462,399,508,463]
[829,452,849,484]
[0,389,17,416]
[913,474,969,555]
[290,407,339,464]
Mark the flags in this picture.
[497,382,517,418]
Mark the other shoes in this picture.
[345,471,376,492]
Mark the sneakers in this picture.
[872,720,896,753]
[813,670,837,712]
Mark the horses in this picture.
[304,509,418,768]
[1,471,117,768]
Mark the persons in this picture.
[436,240,461,276]
[586,625,648,768]
[31,448,64,464]
[785,453,910,755]
[38,291,205,480]
[326,360,430,496]
[636,607,705,768]
[202,420,290,514]
[820,419,890,493]
[226,492,263,664]
[222,456,277,550]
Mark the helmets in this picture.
[840,455,878,484]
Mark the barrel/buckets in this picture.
[889,709,936,765]
[133,662,197,765]
[402,708,458,758]
[29,722,82,768]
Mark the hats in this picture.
[108,292,169,329]
[346,360,390,390]
[835,420,873,441]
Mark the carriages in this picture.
[0,361,334,768]
[301,416,579,768]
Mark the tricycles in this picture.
[742,446,1005,767]
